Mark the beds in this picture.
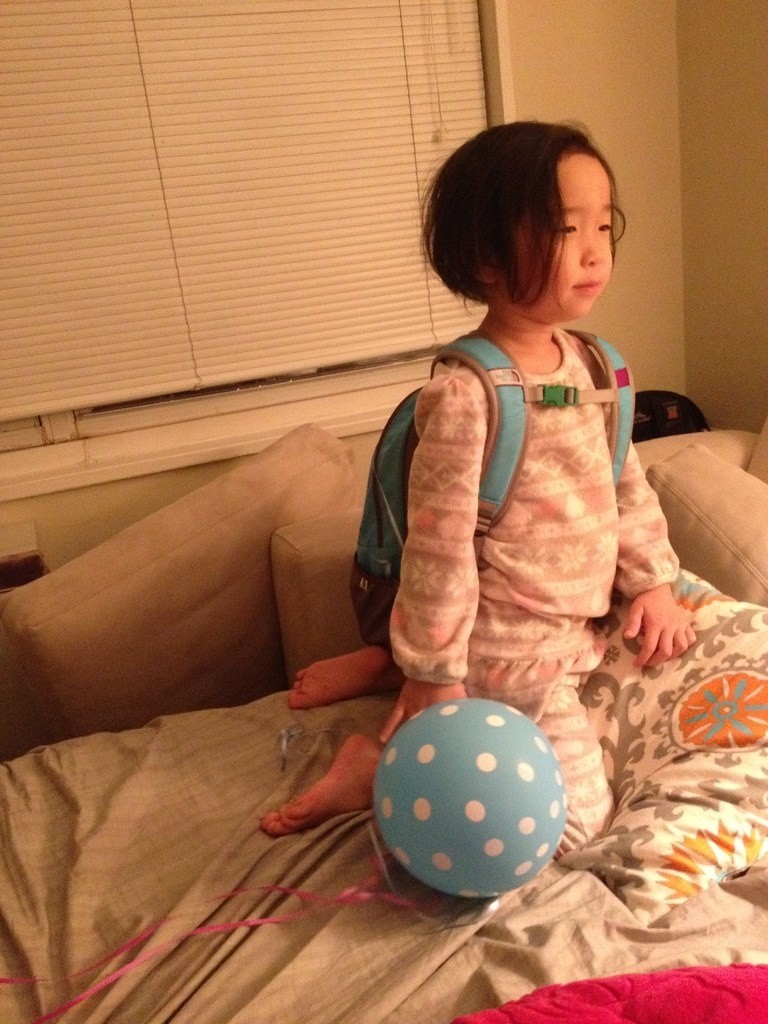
[2,567,768,1023]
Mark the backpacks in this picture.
[349,330,630,647]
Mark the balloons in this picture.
[374,697,567,896]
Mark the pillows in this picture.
[0,422,357,741]
[646,442,767,613]
[264,422,761,688]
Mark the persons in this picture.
[260,118,698,870]
